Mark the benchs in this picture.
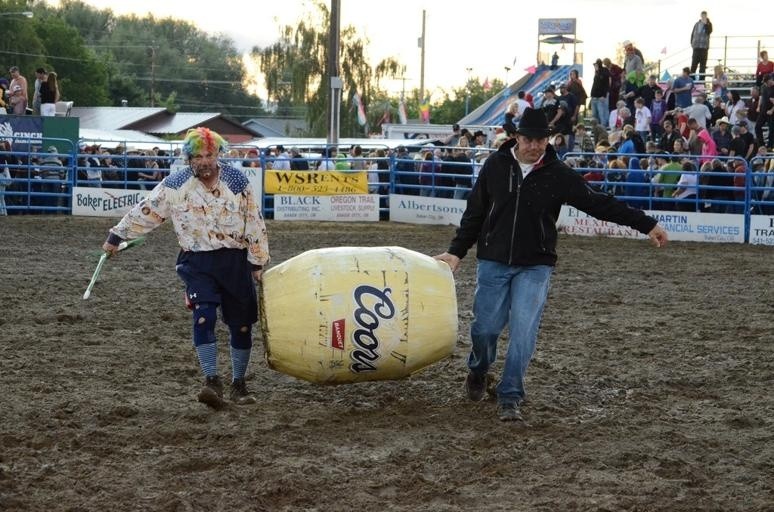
[570,72,774,153]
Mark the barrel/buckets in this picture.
[259,246,459,385]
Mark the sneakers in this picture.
[196,375,225,412]
[463,366,487,402]
[228,378,256,407]
[495,398,524,422]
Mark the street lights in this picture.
[463,66,473,118]
[0,11,35,21]
[120,99,128,107]
[504,64,512,88]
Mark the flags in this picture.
[415,97,430,124]
[349,93,367,127]
[375,102,390,123]
[395,100,407,125]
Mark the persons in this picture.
[434,41,772,212]
[433,108,667,420]
[0,65,60,118]
[103,127,270,410]
[2,139,433,216]
[689,12,711,80]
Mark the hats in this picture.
[122,145,139,155]
[671,106,684,116]
[621,39,634,49]
[715,116,734,128]
[735,119,748,127]
[91,143,102,154]
[501,107,558,139]
